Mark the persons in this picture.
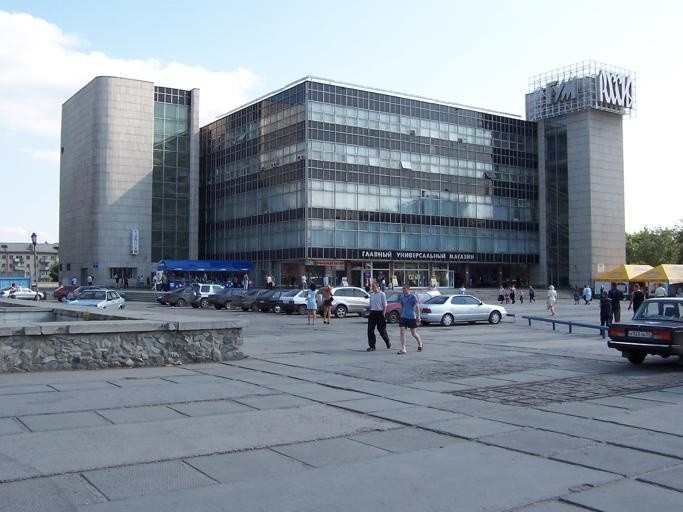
[572,281,667,334]
[497,283,535,305]
[242,273,250,291]
[459,285,466,295]
[8,283,17,300]
[365,277,391,352]
[300,275,335,326]
[397,284,423,355]
[71,272,94,286]
[546,285,558,316]
[114,272,146,290]
[266,272,276,290]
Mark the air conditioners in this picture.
[271,163,275,167]
[298,155,303,160]
[408,130,415,135]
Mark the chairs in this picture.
[665,307,675,317]
[84,293,108,299]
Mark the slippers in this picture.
[398,344,423,354]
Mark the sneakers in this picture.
[367,345,392,351]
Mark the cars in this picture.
[54,285,126,310]
[606,297,682,365]
[0,286,45,301]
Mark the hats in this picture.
[549,285,554,290]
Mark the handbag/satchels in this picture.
[328,297,333,302]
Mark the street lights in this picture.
[31,233,38,301]
